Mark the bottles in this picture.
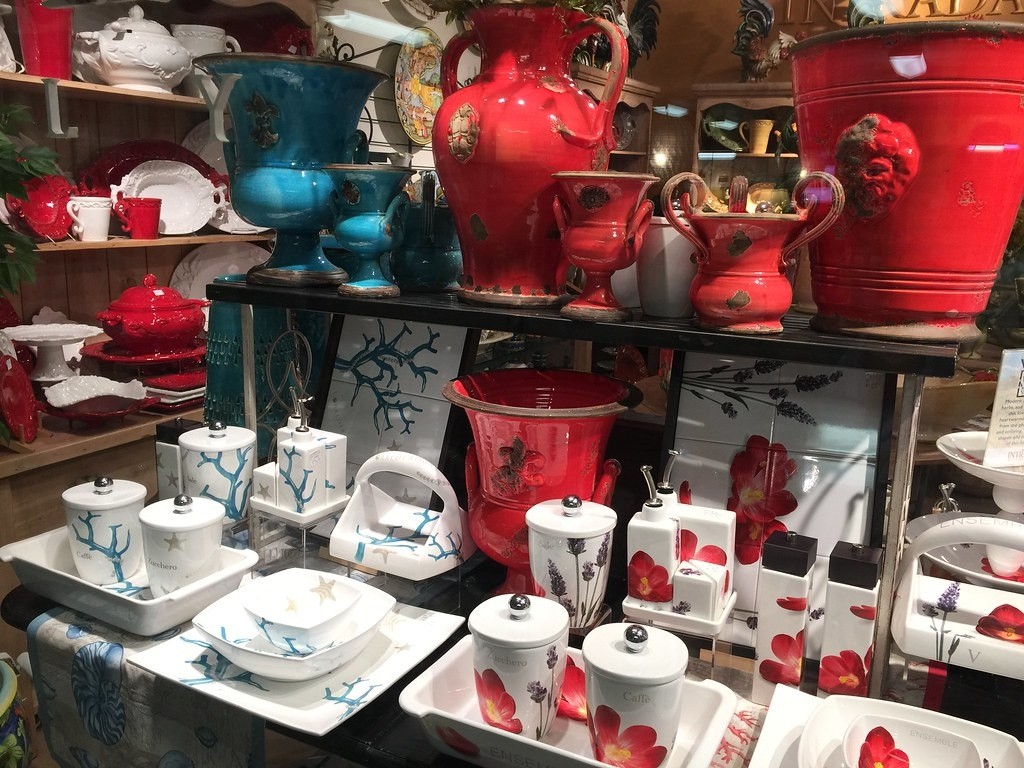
[191,50,463,299]
[203,273,309,461]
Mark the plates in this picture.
[125,601,466,736]
[168,243,272,333]
[394,26,445,145]
[748,683,1024,768]
[110,160,225,236]
[181,115,272,235]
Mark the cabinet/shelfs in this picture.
[1,277,960,768]
[568,63,661,176]
[1,0,316,662]
[689,81,800,212]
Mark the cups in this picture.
[169,23,242,99]
[635,198,696,319]
[178,419,257,525]
[524,494,617,629]
[115,191,162,240]
[581,622,689,768]
[14,0,73,81]
[61,477,148,586]
[467,593,570,742]
[139,494,226,598]
[66,196,113,242]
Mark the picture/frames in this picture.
[656,348,898,673]
[283,312,482,548]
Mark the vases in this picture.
[550,21,1024,336]
[191,52,463,297]
[439,368,643,597]
[433,8,628,309]
[0,659,33,768]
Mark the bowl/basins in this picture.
[191,566,398,684]
[905,511,1024,595]
[796,694,1024,768]
[0,525,260,637]
[935,431,1024,491]
[398,634,737,768]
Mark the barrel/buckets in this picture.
[786,19,1024,345]
